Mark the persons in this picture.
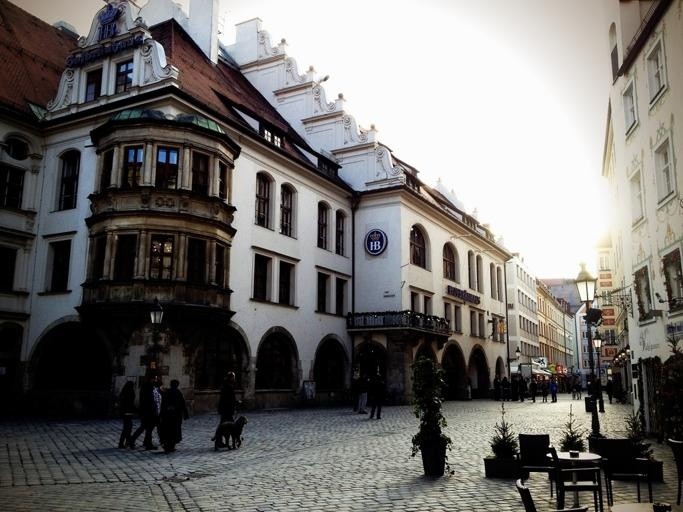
[358,374,370,414]
[606,379,614,404]
[494,372,582,402]
[370,373,384,420]
[214,371,241,447]
[118,372,189,454]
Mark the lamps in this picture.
[507,347,521,363]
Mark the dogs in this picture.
[211,415,248,450]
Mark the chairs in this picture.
[516,434,683,512]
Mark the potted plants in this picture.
[409,358,455,482]
[484,399,520,481]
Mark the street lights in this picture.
[148,294,165,387]
[514,345,524,401]
[594,327,605,412]
[577,261,605,437]
